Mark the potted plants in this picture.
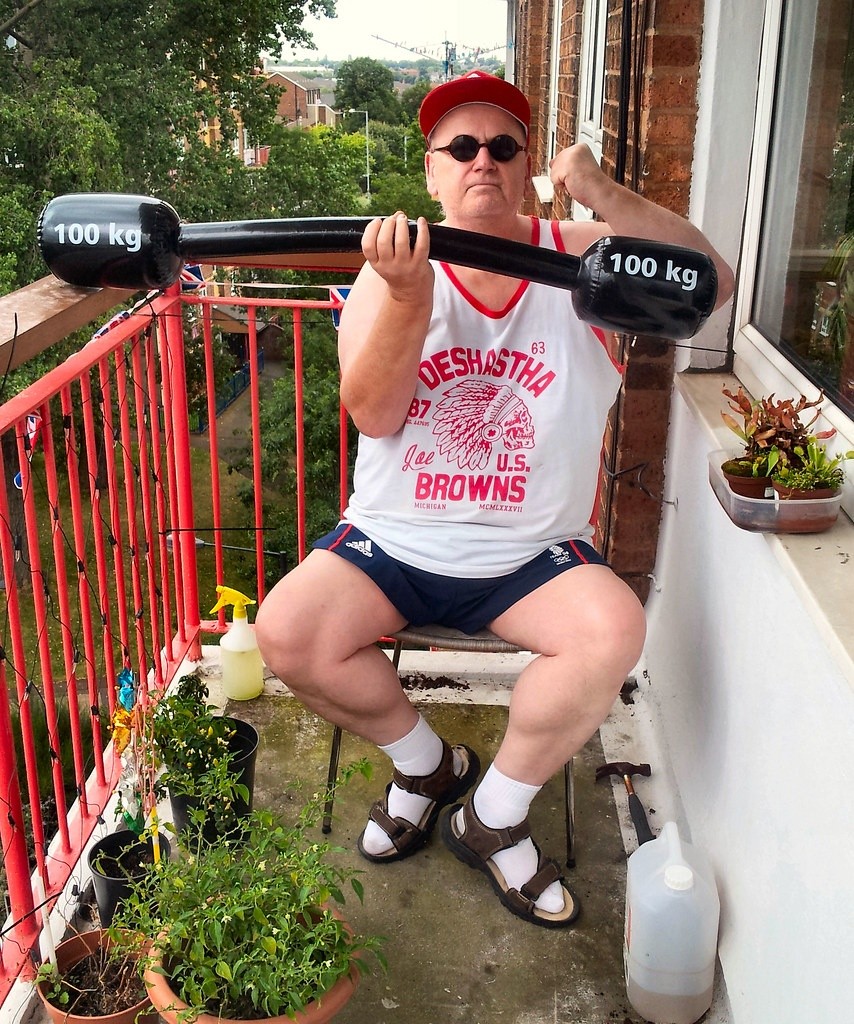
[720,383,824,517]
[771,434,854,533]
[10,901,158,1024]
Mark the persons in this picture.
[254,69,736,928]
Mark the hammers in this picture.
[595,761,657,846]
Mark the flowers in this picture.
[105,664,249,833]
[102,752,389,1024]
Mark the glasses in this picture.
[429,134,528,162]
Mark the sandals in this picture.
[357,736,480,863]
[437,793,581,929]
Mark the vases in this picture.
[165,716,260,856]
[143,901,363,1024]
[86,827,171,931]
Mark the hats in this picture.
[418,71,532,148]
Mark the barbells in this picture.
[34,190,718,343]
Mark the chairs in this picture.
[323,624,577,871]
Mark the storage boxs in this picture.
[706,447,844,534]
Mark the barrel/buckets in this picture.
[626,823,720,1024]
